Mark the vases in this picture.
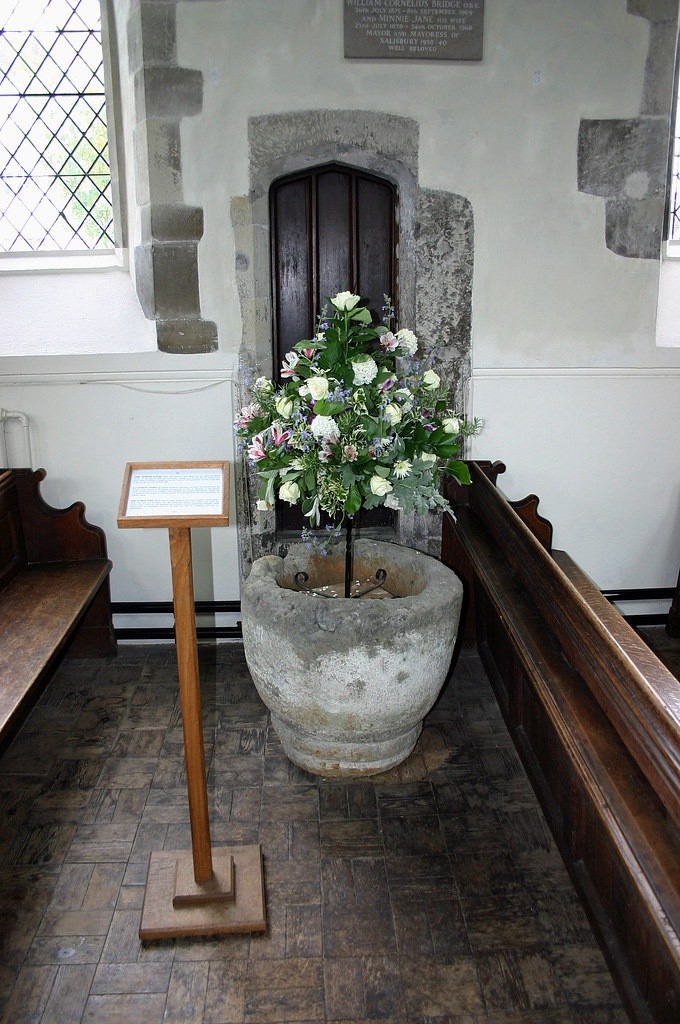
[240,538,462,777]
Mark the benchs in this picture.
[0,467,115,762]
[442,461,680,1024]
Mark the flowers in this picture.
[232,291,489,599]
